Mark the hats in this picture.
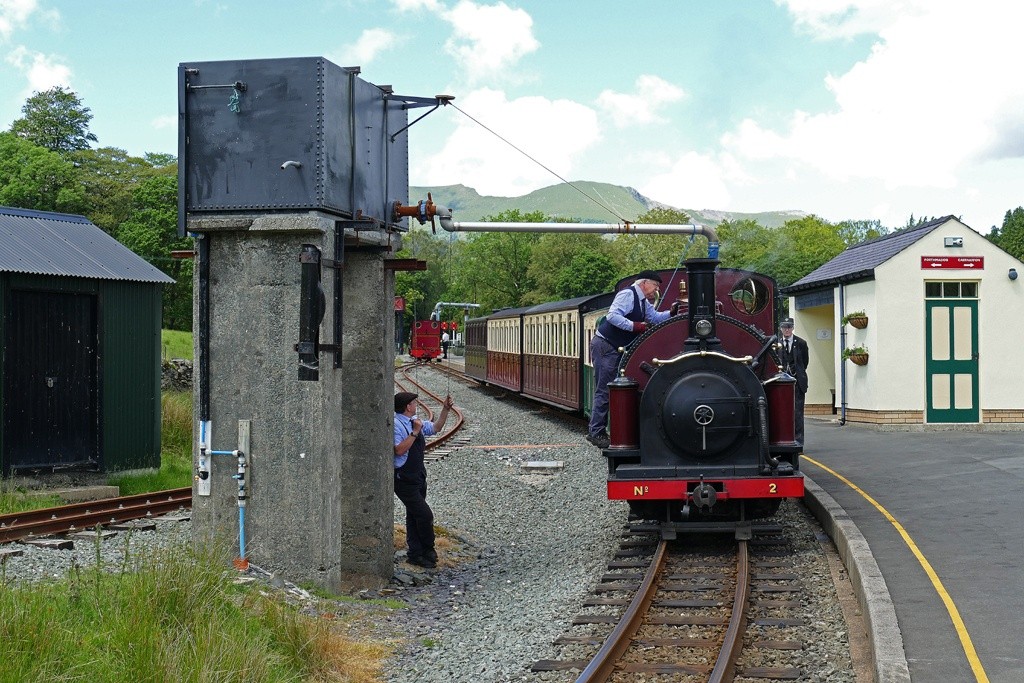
[394,392,418,413]
[779,318,795,327]
[636,269,663,283]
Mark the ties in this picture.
[784,340,789,353]
[642,299,645,321]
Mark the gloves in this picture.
[634,322,645,333]
[670,302,680,316]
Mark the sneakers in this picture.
[585,432,610,449]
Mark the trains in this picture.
[465,257,805,542]
[410,320,442,361]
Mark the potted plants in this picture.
[841,309,868,330]
[841,343,869,366]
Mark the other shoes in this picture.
[406,551,438,568]
[794,440,804,447]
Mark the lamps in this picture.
[1008,268,1018,280]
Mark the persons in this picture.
[442,331,449,359]
[393,392,454,568]
[586,270,679,448]
[777,318,809,446]
